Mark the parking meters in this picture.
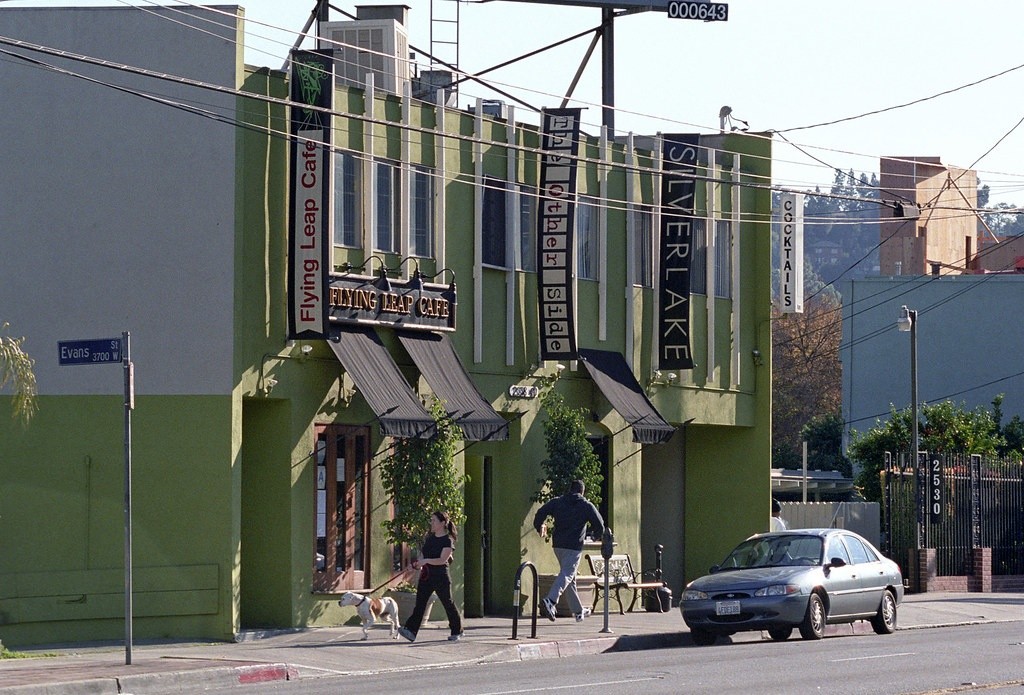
[598,527,615,634]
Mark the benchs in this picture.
[585,553,667,615]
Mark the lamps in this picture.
[344,256,456,303]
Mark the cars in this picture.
[678,527,905,645]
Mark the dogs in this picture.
[337,589,403,640]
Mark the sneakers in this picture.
[543,597,557,621]
[575,607,591,622]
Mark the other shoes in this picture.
[398,627,416,643]
[448,631,465,641]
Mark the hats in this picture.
[772,498,781,513]
[570,479,585,493]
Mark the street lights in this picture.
[897,305,920,593]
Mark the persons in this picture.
[773,542,792,561]
[534,480,604,622]
[398,512,464,642]
[772,499,785,531]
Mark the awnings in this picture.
[311,325,460,459]
[579,349,693,466]
[393,329,529,458]
